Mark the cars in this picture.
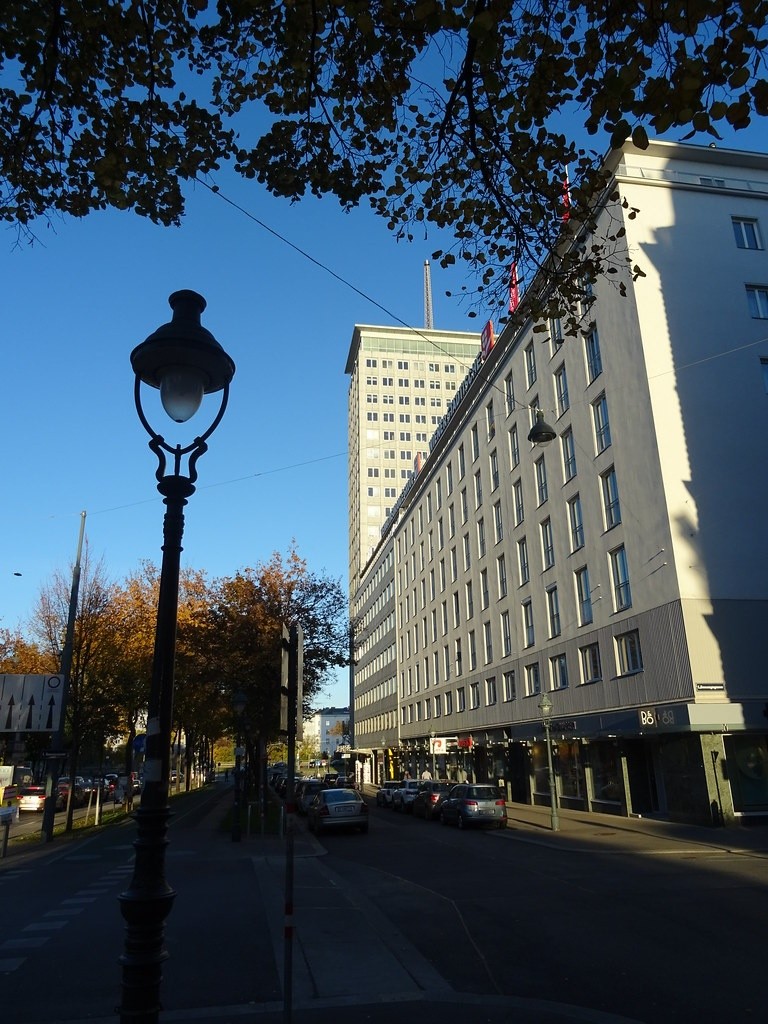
[16,752,186,814]
[266,761,509,832]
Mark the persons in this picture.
[224,770,228,782]
[422,768,432,781]
[404,770,412,781]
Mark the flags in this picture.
[507,262,519,312]
[560,177,571,224]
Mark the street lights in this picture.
[128,290,236,986]
[538,690,563,832]
